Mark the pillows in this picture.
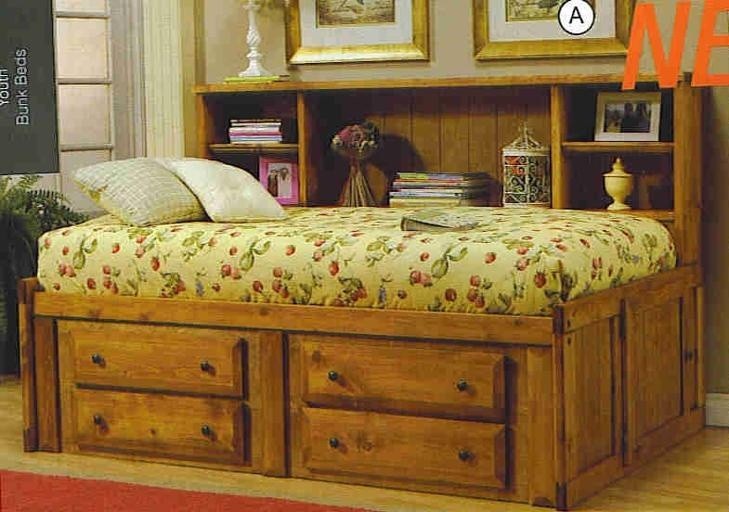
[157,156,288,223]
[71,155,206,227]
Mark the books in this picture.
[228,118,289,146]
[385,170,489,206]
[400,206,480,234]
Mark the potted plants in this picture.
[0,173,90,375]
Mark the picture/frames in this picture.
[280,0,435,72]
[259,155,300,206]
[594,89,662,143]
[468,0,642,62]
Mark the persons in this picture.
[268,167,290,197]
[603,101,651,133]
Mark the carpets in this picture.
[1,467,388,512]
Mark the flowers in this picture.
[327,113,381,208]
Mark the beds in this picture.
[16,71,704,512]
